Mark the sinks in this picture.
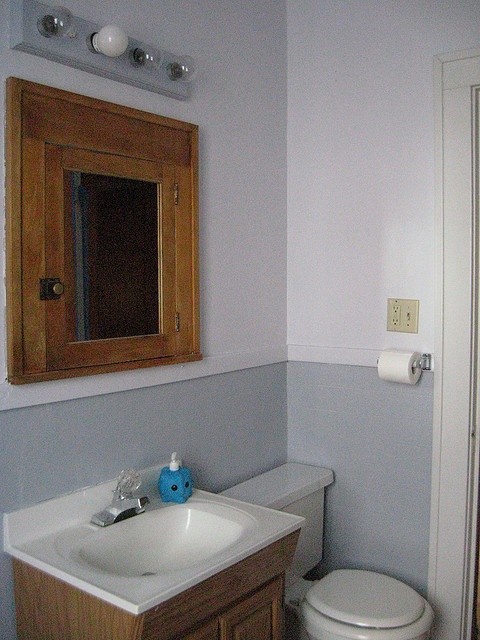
[78,505,244,577]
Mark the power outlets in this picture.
[386,297,428,331]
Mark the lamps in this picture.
[85,22,130,59]
[131,40,164,70]
[167,54,195,78]
[37,3,73,38]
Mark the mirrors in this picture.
[5,74,200,385]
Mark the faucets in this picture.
[90,468,150,527]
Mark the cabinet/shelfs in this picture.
[12,528,300,639]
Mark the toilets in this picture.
[217,460,435,640]
